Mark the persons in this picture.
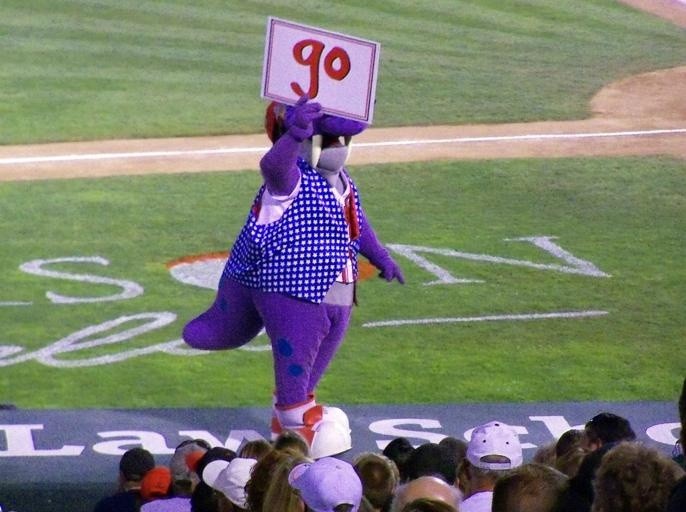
[93,377,685,512]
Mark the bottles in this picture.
[118,447,156,482]
[288,455,363,512]
[138,464,169,500]
[464,421,525,471]
[170,442,208,492]
[200,453,264,511]
